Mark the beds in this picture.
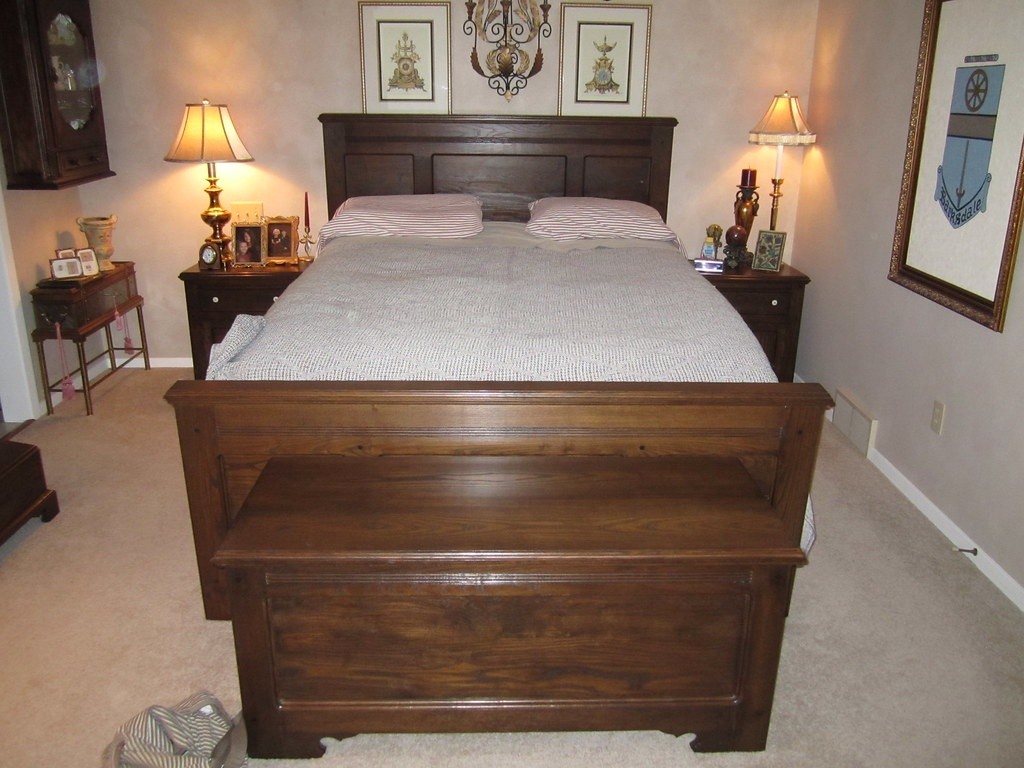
[163,113,836,760]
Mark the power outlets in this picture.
[930,400,946,435]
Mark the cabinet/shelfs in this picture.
[0,0,117,190]
[29,261,151,416]
[688,260,812,383]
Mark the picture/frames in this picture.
[358,0,452,115]
[48,257,84,281]
[54,247,76,259]
[74,247,101,277]
[230,221,266,269]
[886,0,1024,333]
[556,2,652,117]
[260,215,299,266]
[751,229,787,272]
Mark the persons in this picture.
[271,228,288,253]
[236,229,260,262]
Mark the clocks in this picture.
[198,239,221,271]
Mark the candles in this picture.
[741,165,757,186]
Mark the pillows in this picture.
[524,196,678,242]
[319,193,484,239]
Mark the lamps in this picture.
[164,98,255,268]
[748,91,817,231]
[462,0,552,103]
